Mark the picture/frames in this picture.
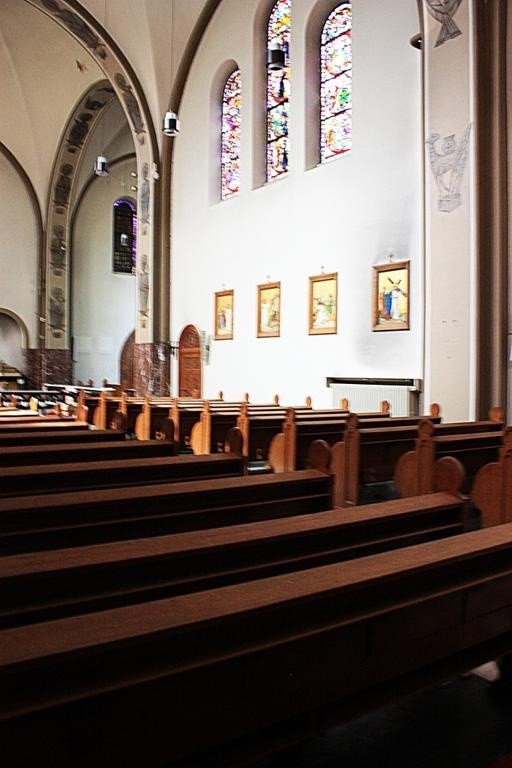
[255,280,282,339]
[306,272,338,336]
[213,289,234,341]
[371,260,410,332]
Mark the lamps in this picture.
[94,0,110,177]
[266,43,285,71]
[162,0,181,137]
